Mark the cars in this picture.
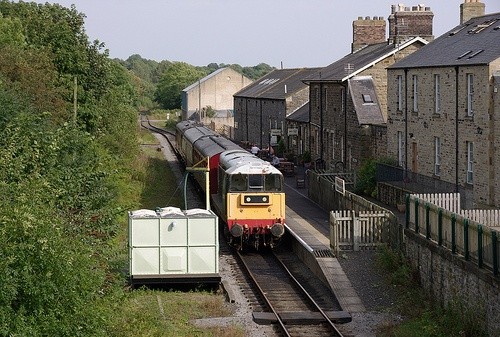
[271,158,295,175]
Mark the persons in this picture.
[251,143,260,155]
[266,145,276,162]
[270,155,279,167]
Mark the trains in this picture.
[174,120,287,252]
